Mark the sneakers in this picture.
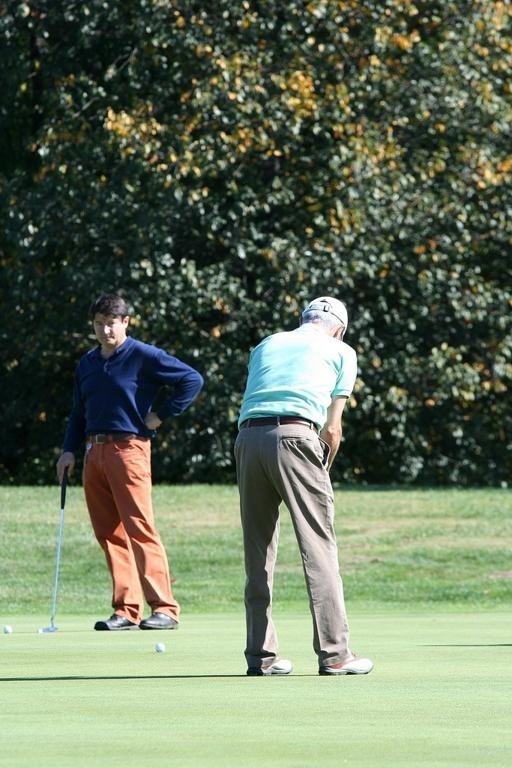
[246,656,293,676]
[318,652,374,676]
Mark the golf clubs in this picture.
[39,466,69,634]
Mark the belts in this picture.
[239,416,318,435]
[86,432,147,443]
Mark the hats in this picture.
[302,296,349,342]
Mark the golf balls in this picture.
[155,643,166,652]
[4,625,13,633]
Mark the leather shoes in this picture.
[94,613,139,632]
[138,612,179,631]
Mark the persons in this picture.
[225,290,376,677]
[53,290,209,633]
